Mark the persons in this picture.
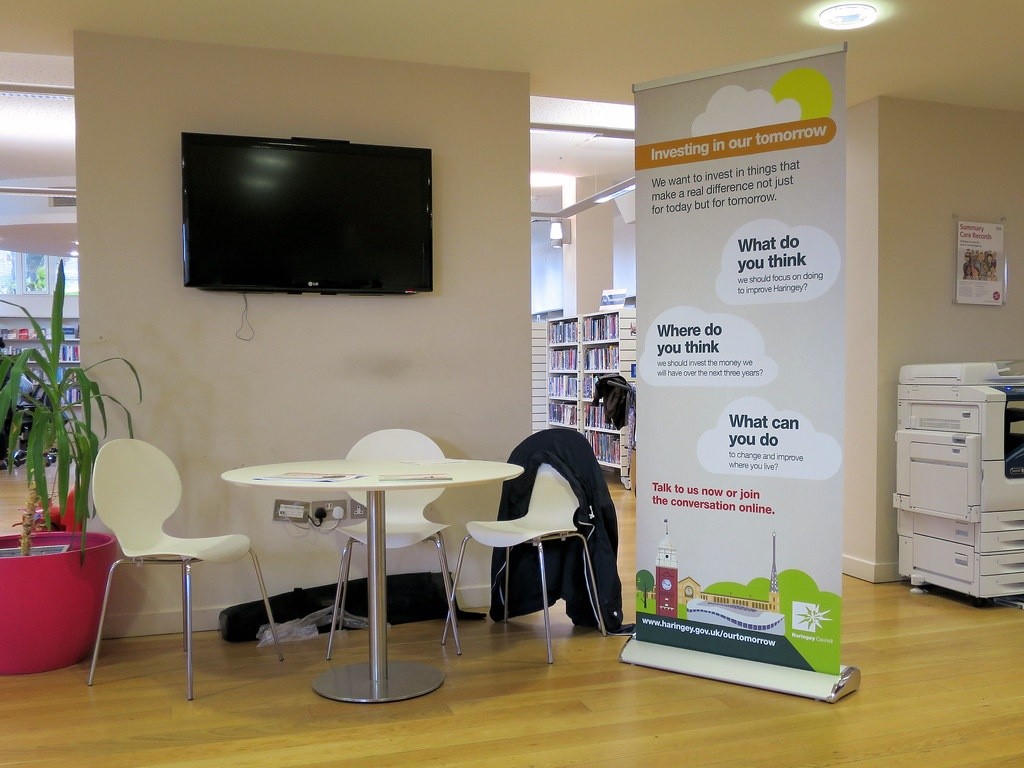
[0,337,14,470]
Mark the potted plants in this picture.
[0,257,142,676]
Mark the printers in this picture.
[891,360,1023,601]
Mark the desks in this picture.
[221,457,525,704]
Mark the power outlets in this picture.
[311,499,347,522]
[350,498,368,518]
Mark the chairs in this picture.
[326,428,461,655]
[89,438,283,700]
[440,428,635,663]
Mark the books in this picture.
[548,314,620,463]
[0,324,81,404]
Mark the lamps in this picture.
[548,216,571,246]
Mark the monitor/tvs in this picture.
[180,129,435,297]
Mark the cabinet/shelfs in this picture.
[1,334,82,409]
[546,307,636,492]
